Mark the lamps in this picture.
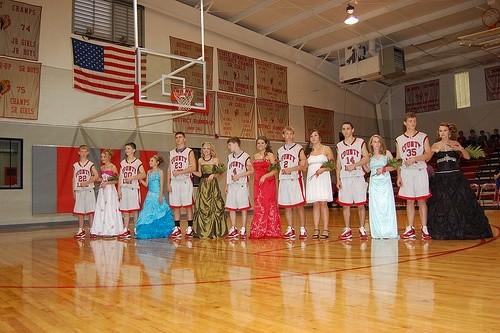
[344,2,359,25]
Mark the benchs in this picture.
[390,151,485,202]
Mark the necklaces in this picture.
[104,163,111,167]
[313,146,319,151]
[202,155,212,162]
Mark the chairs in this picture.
[469,183,500,208]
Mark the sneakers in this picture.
[169,226,182,237]
[299,227,308,239]
[282,226,295,238]
[185,226,195,238]
[338,227,352,239]
[239,227,247,239]
[225,226,239,238]
[75,227,86,238]
[89,228,96,237]
[358,227,368,240]
[401,226,416,239]
[118,227,131,238]
[133,228,136,234]
[421,226,432,240]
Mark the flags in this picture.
[70,37,147,100]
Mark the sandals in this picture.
[312,229,320,239]
[320,230,329,238]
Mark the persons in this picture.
[361,135,401,239]
[456,129,500,193]
[395,112,432,240]
[300,129,334,241]
[90,149,123,236]
[73,145,98,238]
[225,136,254,238]
[135,154,175,239]
[167,131,196,238]
[189,141,228,239]
[276,127,308,239]
[424,122,493,240]
[247,136,282,239]
[117,142,146,237]
[336,122,369,239]
